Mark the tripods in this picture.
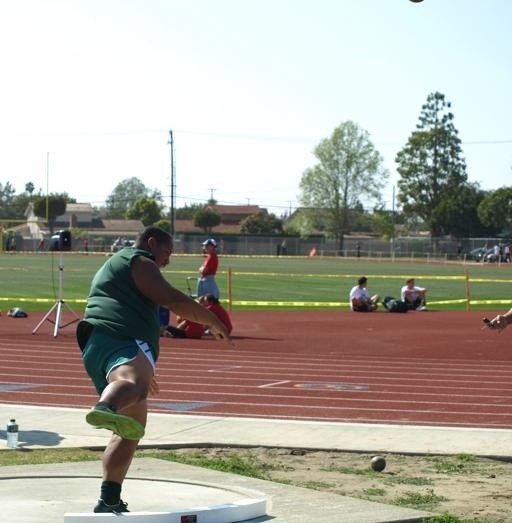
[32,250,81,339]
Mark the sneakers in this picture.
[84,402,144,442]
[92,501,130,514]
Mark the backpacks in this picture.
[383,295,408,312]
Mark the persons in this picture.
[75,225,235,512]
[350,276,379,311]
[481,307,512,333]
[158,304,170,337]
[39,238,48,250]
[163,319,204,339]
[198,295,211,335]
[400,278,428,310]
[84,239,88,255]
[479,241,512,262]
[197,236,220,301]
[282,238,288,255]
[12,236,16,251]
[204,293,232,338]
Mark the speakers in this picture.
[50,229,71,250]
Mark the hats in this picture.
[203,238,218,249]
